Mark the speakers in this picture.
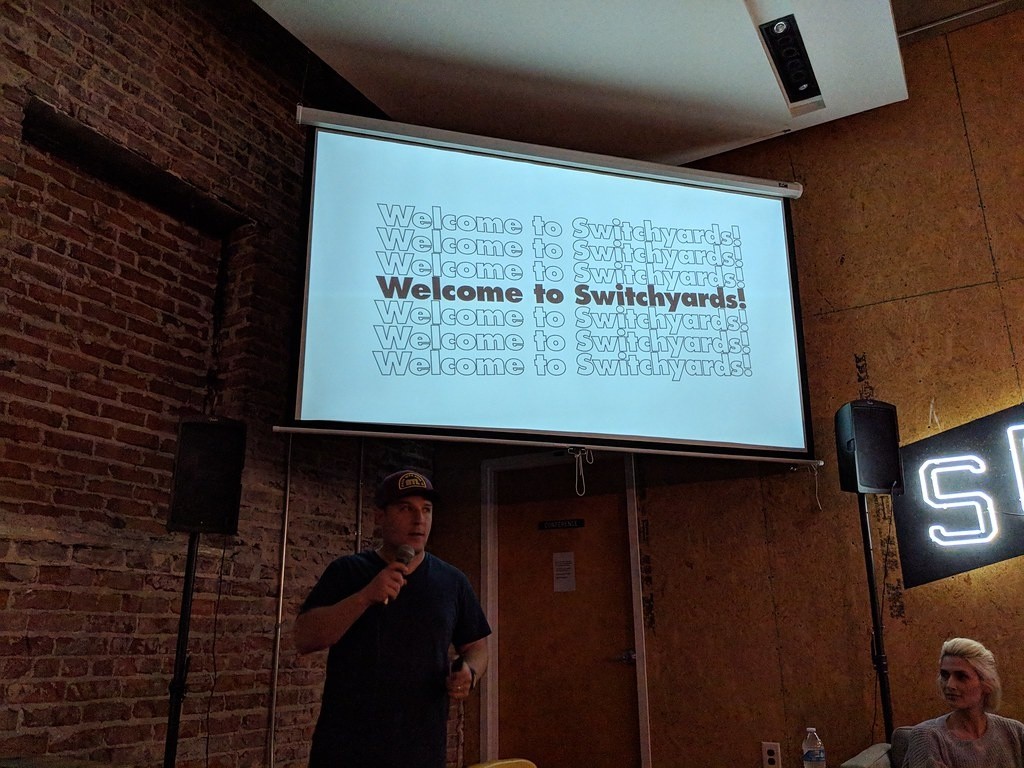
[833,398,906,495]
[166,418,247,536]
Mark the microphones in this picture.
[382,544,415,607]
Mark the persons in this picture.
[291,468,495,768]
[902,637,1024,768]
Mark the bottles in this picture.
[802,728,825,768]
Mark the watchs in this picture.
[470,668,477,689]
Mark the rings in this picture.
[457,686,461,692]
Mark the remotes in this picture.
[451,655,463,672]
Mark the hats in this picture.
[375,470,433,509]
